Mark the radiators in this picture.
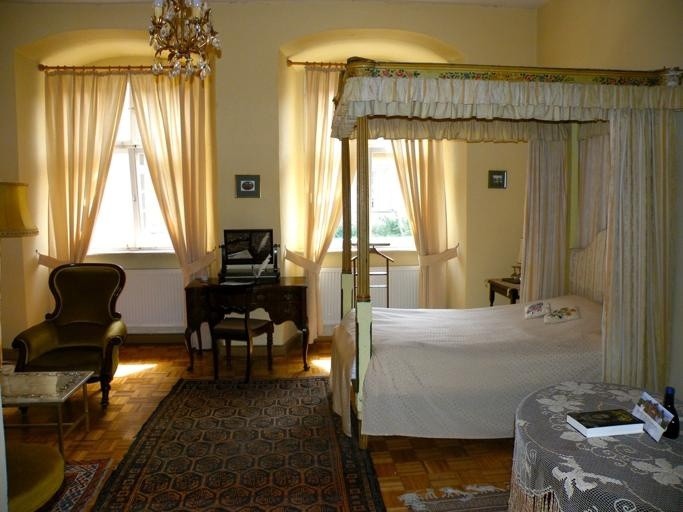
[115,269,189,334]
[317,267,422,337]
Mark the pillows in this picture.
[549,293,602,335]
[543,306,581,324]
[523,298,550,318]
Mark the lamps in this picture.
[145,0,224,79]
[1,182,39,238]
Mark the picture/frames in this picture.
[487,169,507,190]
[232,173,261,199]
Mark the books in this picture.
[566,409,646,439]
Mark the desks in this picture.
[181,278,312,370]
[508,381,682,511]
[486,277,521,306]
[1,366,94,459]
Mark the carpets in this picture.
[397,478,512,510]
[38,450,109,512]
[88,375,388,512]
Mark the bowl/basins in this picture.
[0,360,17,375]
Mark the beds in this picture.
[329,54,683,454]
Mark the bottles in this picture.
[663,386,679,441]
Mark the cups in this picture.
[201,269,208,283]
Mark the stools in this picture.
[201,278,273,381]
[0,436,65,511]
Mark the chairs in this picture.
[12,261,127,419]
